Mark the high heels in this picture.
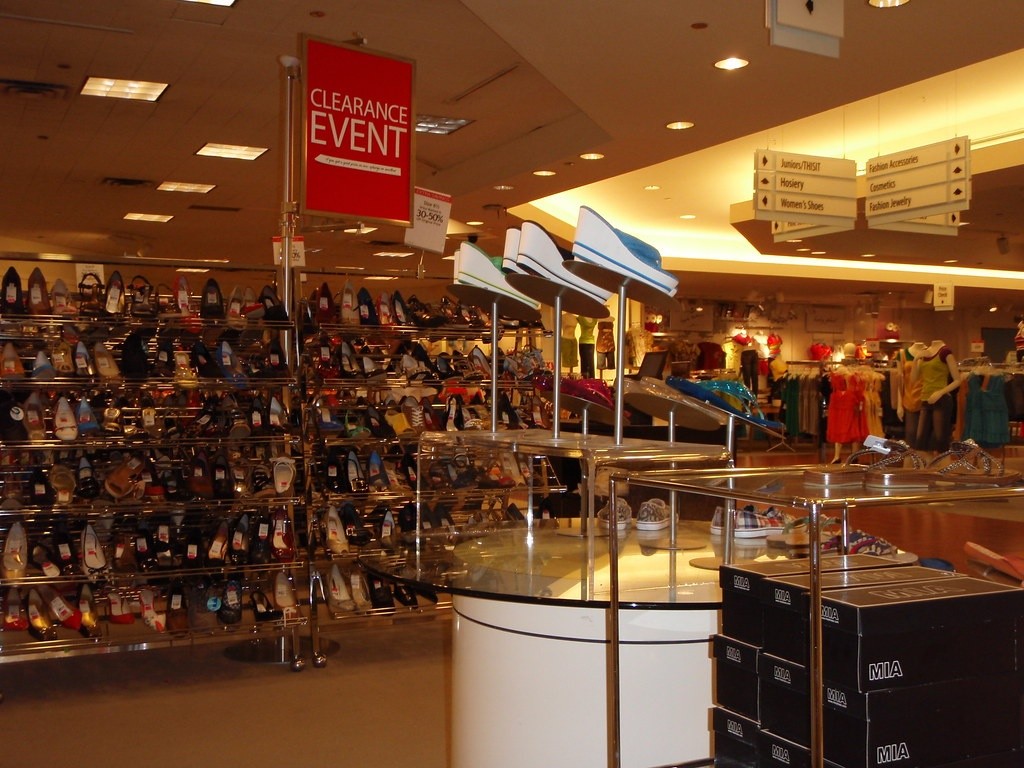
[501,227,527,275]
[454,241,541,310]
[571,206,680,297]
[517,219,613,304]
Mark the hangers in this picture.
[783,359,896,381]
[959,356,1024,380]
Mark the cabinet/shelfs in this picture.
[0,250,567,672]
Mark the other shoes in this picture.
[0,266,554,642]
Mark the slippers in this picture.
[786,517,853,549]
[665,375,786,432]
[790,529,897,556]
[862,439,1024,493]
[766,512,829,547]
[801,439,927,486]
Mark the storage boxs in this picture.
[712,553,1024,768]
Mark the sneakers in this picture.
[709,502,764,536]
[635,498,679,530]
[734,506,798,538]
[598,498,631,530]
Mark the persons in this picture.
[1014,320,1024,362]
[807,343,832,360]
[897,342,928,448]
[561,313,578,367]
[753,330,768,357]
[910,340,961,451]
[596,316,616,370]
[767,332,783,356]
[741,350,758,395]
[732,330,752,347]
[770,356,787,382]
[823,343,859,464]
[576,316,597,379]
